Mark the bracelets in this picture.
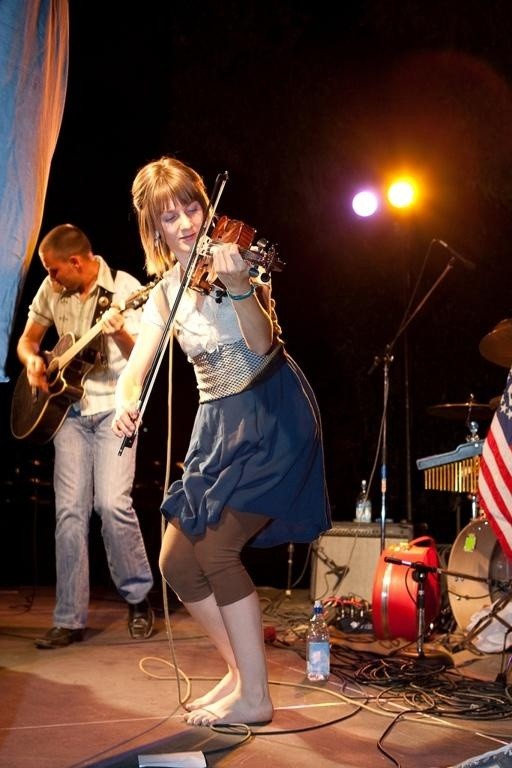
[224,284,255,302]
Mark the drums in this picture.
[446,517,512,655]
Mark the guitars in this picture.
[11,280,161,443]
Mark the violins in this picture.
[188,215,286,295]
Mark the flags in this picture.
[477,368,512,563]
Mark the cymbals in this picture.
[480,326,512,369]
[431,402,489,414]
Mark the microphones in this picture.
[438,244,478,275]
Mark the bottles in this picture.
[355,479,372,524]
[305,600,331,682]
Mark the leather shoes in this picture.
[127,598,155,639]
[34,626,86,650]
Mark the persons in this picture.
[16,223,154,650]
[108,156,333,728]
